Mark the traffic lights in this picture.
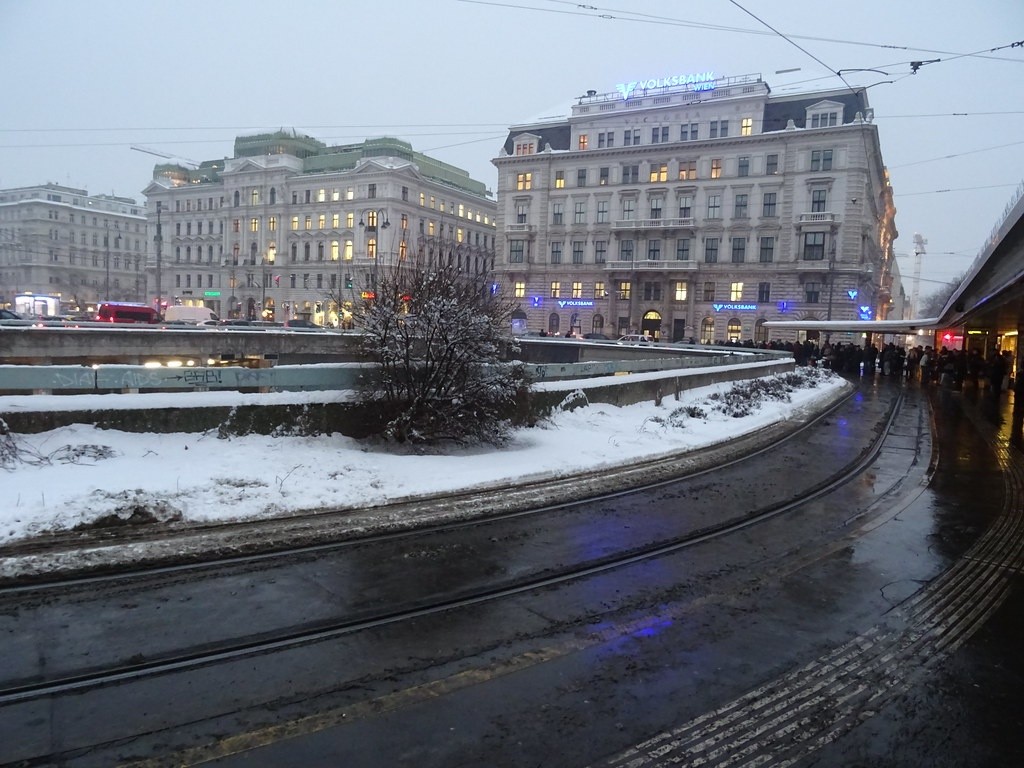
[347,281,352,289]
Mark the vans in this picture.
[94,303,161,326]
[163,306,219,324]
[617,334,655,347]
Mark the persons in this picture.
[565,331,571,337]
[539,329,545,336]
[715,338,820,367]
[689,337,695,344]
[821,339,906,376]
[906,345,1013,393]
[706,339,711,345]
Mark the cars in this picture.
[0,309,25,325]
[31,315,78,328]
[285,319,324,333]
[195,319,220,329]
[215,319,260,331]
[158,321,191,330]
[579,333,615,344]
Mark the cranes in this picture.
[129,144,201,167]
[877,236,955,322]
